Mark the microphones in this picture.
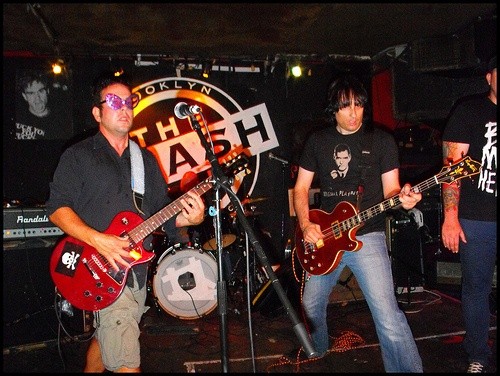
[268,153,289,165]
[337,280,352,289]
[173,102,201,119]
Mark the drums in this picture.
[147,240,222,320]
[201,233,246,273]
[239,197,276,215]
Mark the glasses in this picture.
[99,93,140,110]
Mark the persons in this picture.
[178,170,237,297]
[293,75,424,373]
[45,77,205,374]
[8,72,73,141]
[322,143,360,212]
[441,55,496,374]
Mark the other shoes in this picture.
[467,361,487,374]
[290,348,308,358]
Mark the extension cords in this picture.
[397,287,424,293]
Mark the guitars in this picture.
[50,152,251,310]
[294,151,485,277]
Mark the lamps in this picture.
[289,57,308,79]
[51,55,66,76]
[110,56,125,77]
[199,58,213,78]
[176,60,189,72]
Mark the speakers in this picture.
[331,266,365,302]
[436,247,499,290]
